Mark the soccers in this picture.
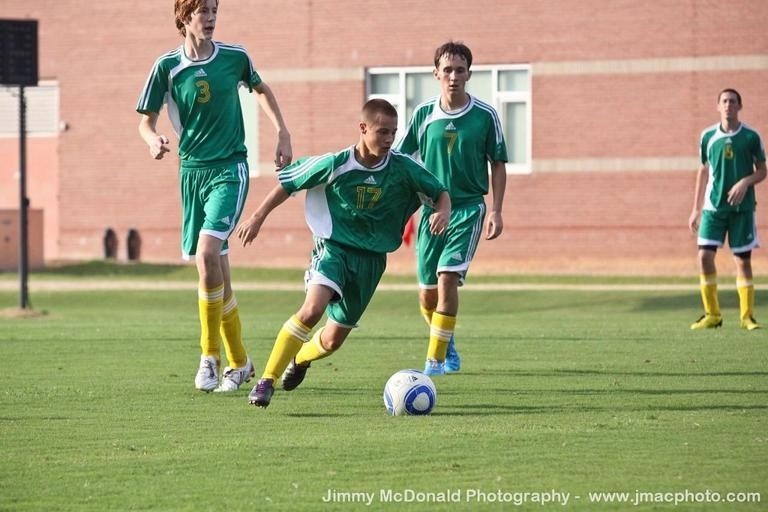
[383,368,435,416]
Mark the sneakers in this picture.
[195,354,220,391]
[690,312,723,330]
[213,354,255,392]
[445,334,460,373]
[741,315,760,330]
[423,358,444,377]
[281,355,311,392]
[247,377,274,407]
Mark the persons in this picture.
[391,42,509,377]
[235,99,452,410]
[134,1,293,393]
[687,88,768,331]
